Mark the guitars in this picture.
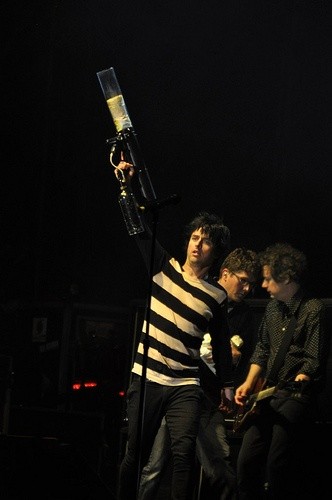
[229,374,305,433]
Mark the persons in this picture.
[234,242,330,500]
[136,247,265,500]
[111,161,230,500]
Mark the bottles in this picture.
[118,185,145,236]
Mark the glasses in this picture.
[227,267,257,289]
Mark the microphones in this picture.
[138,193,181,215]
